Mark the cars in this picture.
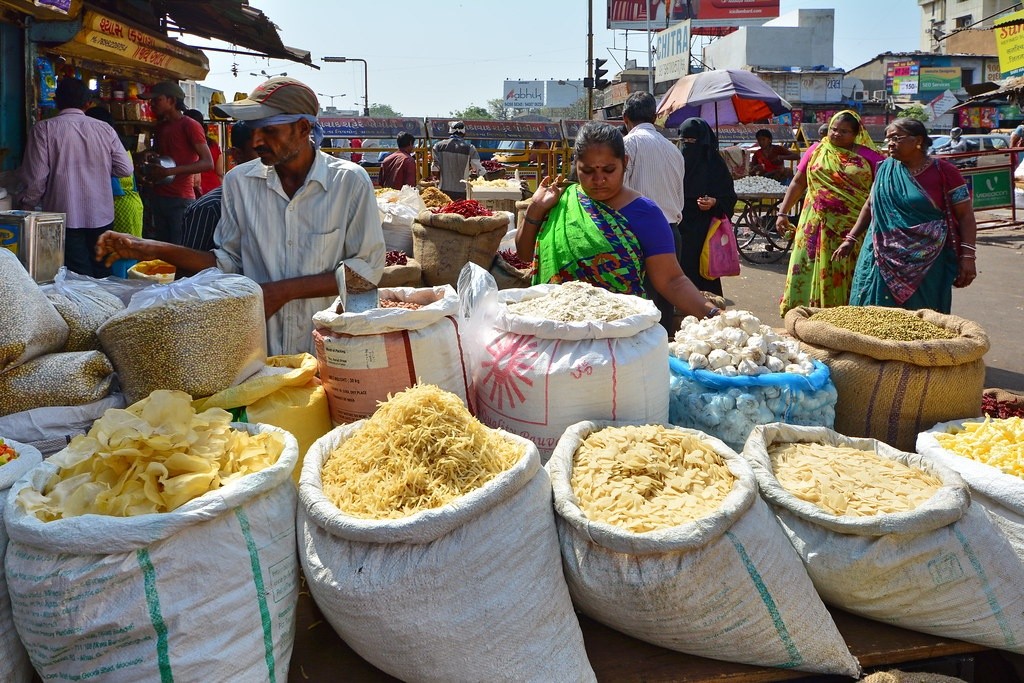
[927,129,1015,156]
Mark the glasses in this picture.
[884,134,910,143]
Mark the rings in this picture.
[971,275,975,279]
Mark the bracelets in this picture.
[843,233,859,243]
[776,213,787,216]
[706,306,719,317]
[961,242,976,260]
[523,211,541,225]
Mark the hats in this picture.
[136,82,185,100]
[212,81,319,121]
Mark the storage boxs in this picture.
[0,210,66,287]
[459,173,523,219]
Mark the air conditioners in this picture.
[873,90,888,101]
[965,20,971,27]
[925,28,931,33]
[854,90,869,102]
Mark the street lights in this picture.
[557,80,579,118]
[318,92,345,105]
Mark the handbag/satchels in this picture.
[700,213,740,280]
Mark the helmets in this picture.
[951,127,963,138]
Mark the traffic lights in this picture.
[595,58,608,89]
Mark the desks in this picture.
[287,566,988,683]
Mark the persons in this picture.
[621,90,684,335]
[319,139,379,166]
[378,132,416,190]
[16,75,144,279]
[1010,125,1024,163]
[430,122,487,200]
[831,117,977,314]
[936,127,967,153]
[175,120,259,281]
[775,110,888,317]
[676,117,738,298]
[97,76,387,355]
[749,129,801,173]
[137,81,223,241]
[653,0,695,19]
[514,120,723,321]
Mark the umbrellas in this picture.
[654,67,793,151]
